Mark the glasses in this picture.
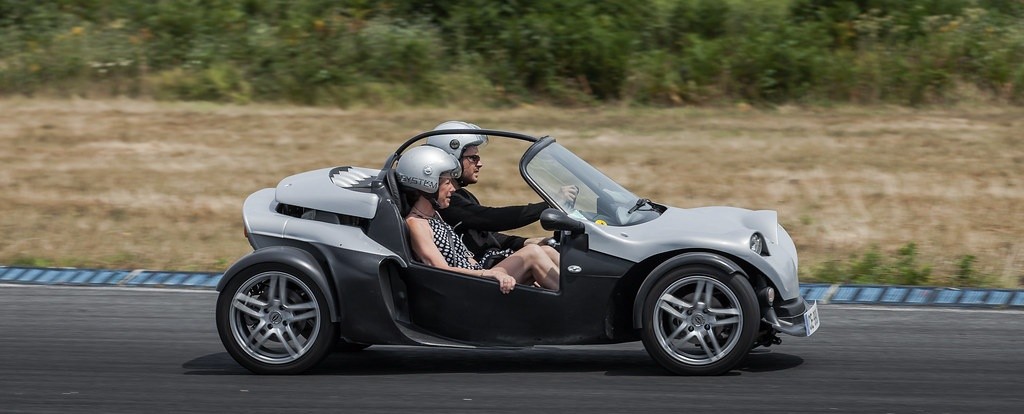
[458,154,481,163]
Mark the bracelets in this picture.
[480,269,485,277]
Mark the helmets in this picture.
[395,145,462,194]
[426,121,488,161]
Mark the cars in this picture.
[213,130,820,376]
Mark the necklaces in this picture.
[413,204,434,218]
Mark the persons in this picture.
[398,119,579,294]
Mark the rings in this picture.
[503,281,507,283]
